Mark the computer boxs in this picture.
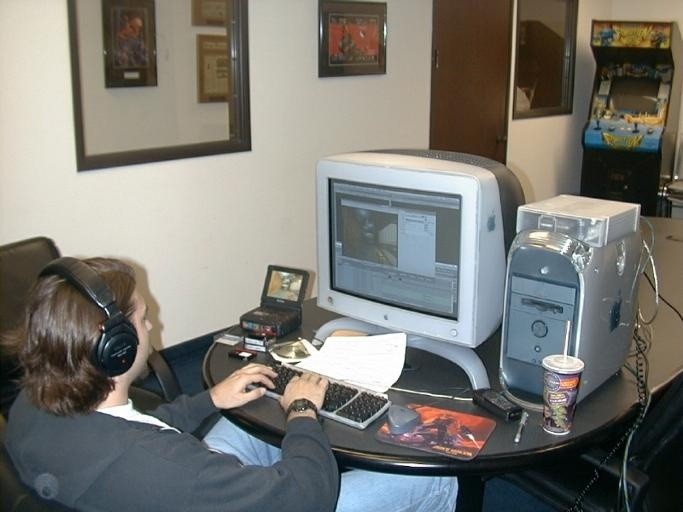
[494,193,641,411]
[239,305,301,336]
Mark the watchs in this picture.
[284,398,319,422]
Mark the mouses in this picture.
[390,404,420,436]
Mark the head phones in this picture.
[36,256,139,376]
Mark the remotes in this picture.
[474,387,522,422]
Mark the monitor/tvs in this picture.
[311,148,526,396]
[605,77,662,113]
[261,265,309,310]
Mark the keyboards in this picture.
[245,363,391,430]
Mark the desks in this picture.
[204,214,683,512]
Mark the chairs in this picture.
[1,238,179,433]
[458,373,683,512]
[0,440,83,512]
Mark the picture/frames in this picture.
[67,1,251,172]
[317,0,387,77]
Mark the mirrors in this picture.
[513,0,578,120]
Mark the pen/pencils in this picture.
[514,411,529,443]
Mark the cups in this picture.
[539,354,585,437]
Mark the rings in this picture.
[318,382,329,390]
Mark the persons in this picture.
[336,24,355,56]
[104,6,153,70]
[267,271,299,304]
[0,256,460,512]
[542,399,557,429]
[356,27,370,55]
